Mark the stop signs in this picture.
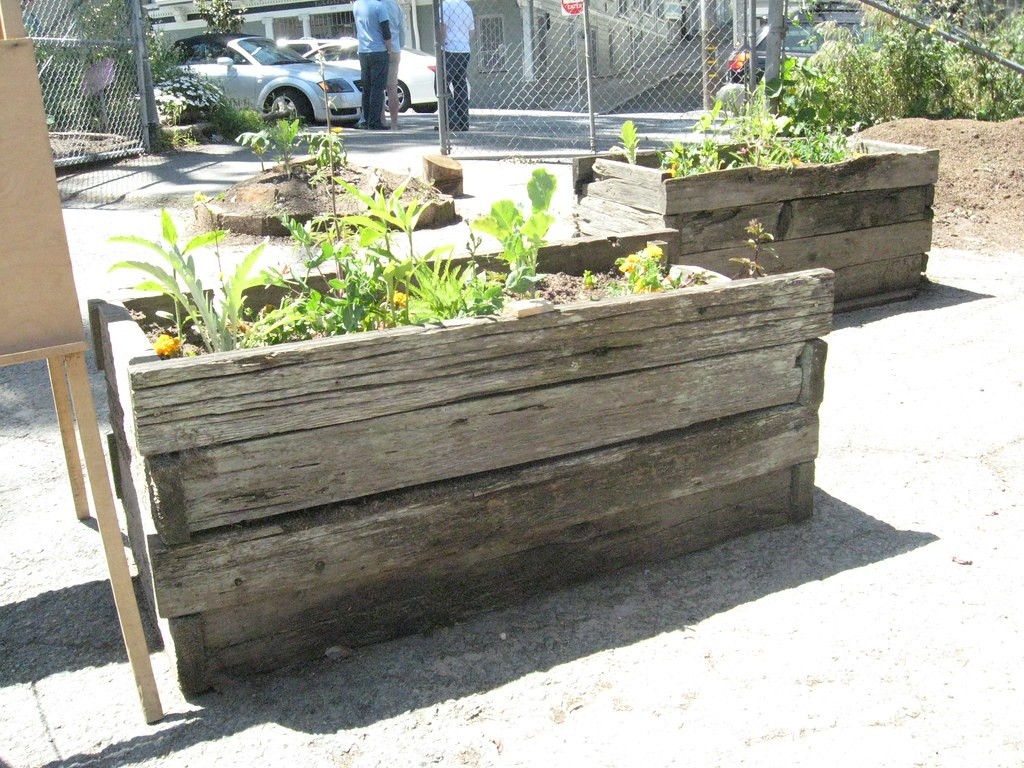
[561,0,590,15]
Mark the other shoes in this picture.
[359,122,391,129]
[434,122,469,132]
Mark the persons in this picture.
[353,0,405,130]
[433,0,475,131]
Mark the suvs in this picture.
[728,8,979,85]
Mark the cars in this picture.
[301,40,471,113]
[277,39,344,61]
[165,33,363,125]
